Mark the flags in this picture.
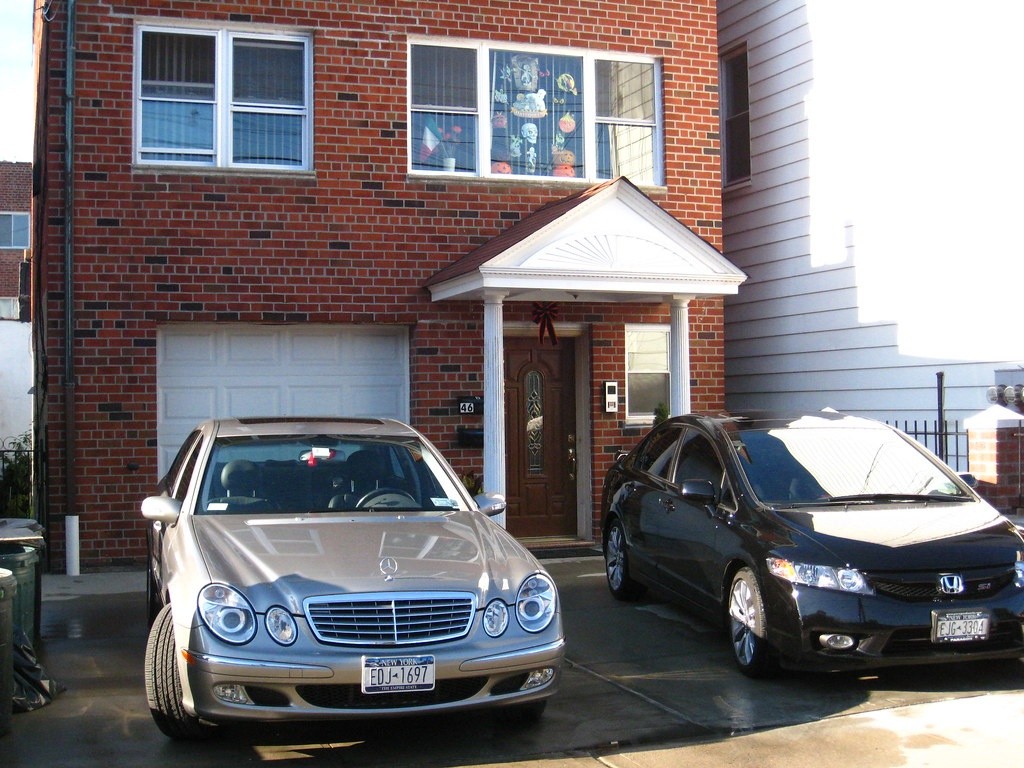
[420,113,443,162]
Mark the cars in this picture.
[600,411,1023,679]
[141,417,567,751]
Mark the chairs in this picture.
[327,449,393,510]
[679,448,723,493]
[210,461,275,511]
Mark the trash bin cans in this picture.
[0,566,20,669]
[0,544,41,652]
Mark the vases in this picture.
[443,159,456,171]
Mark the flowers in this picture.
[437,125,463,157]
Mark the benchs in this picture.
[214,461,360,512]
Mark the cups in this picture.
[443,158,455,172]
